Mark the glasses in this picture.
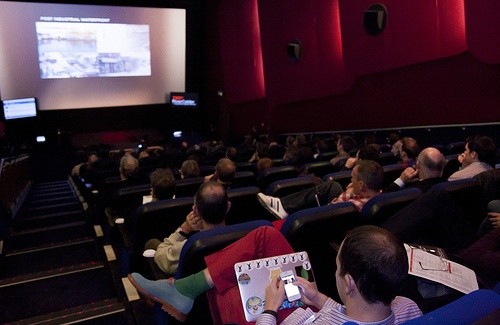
[407,243,451,273]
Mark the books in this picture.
[403,242,479,294]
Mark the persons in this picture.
[211,158,239,190]
[366,130,455,165]
[448,135,495,182]
[329,136,355,168]
[128,224,423,325]
[257,161,382,221]
[0,198,12,254]
[381,147,448,193]
[328,187,500,290]
[144,182,231,282]
[174,161,200,180]
[71,132,337,196]
[340,145,380,171]
[105,169,176,227]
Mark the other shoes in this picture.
[105,207,115,226]
[142,274,167,281]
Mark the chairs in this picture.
[78,143,500,325]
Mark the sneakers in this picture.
[257,192,288,219]
[128,272,194,322]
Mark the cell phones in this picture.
[279,270,301,302]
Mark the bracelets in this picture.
[261,310,278,321]
[177,226,188,237]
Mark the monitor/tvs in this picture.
[1,97,37,120]
[170,92,198,108]
